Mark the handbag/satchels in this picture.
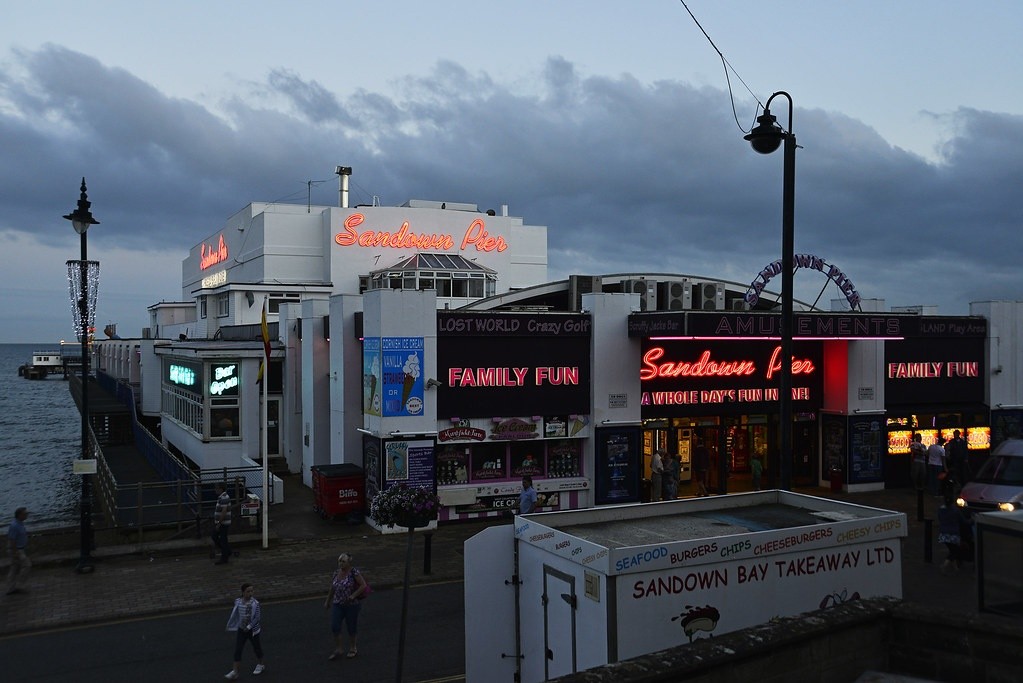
[351,568,372,598]
[750,464,755,477]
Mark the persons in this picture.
[693,437,710,497]
[323,552,367,660]
[949,430,971,483]
[908,433,925,490]
[225,583,265,680]
[516,476,537,514]
[938,497,975,561]
[928,437,946,480]
[650,448,682,502]
[5,507,28,595]
[211,482,231,565]
[748,455,762,491]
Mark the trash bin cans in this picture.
[311,463,368,522]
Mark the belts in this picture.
[6,547,25,550]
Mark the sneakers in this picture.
[225,671,241,680]
[253,664,265,674]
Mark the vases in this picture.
[393,504,431,527]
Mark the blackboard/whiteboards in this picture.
[594,425,643,506]
[821,411,848,483]
[848,414,885,483]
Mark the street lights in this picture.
[742,91,798,490]
[62,178,100,576]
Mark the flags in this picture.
[255,302,271,385]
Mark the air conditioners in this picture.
[698,282,725,311]
[664,281,692,309]
[569,274,603,313]
[732,298,745,310]
[620,279,657,313]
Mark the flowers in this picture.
[364,478,444,530]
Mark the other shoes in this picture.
[346,649,358,658]
[749,487,761,491]
[703,493,711,497]
[215,558,227,565]
[694,493,702,496]
[328,650,344,660]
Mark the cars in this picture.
[951,437,1023,521]
